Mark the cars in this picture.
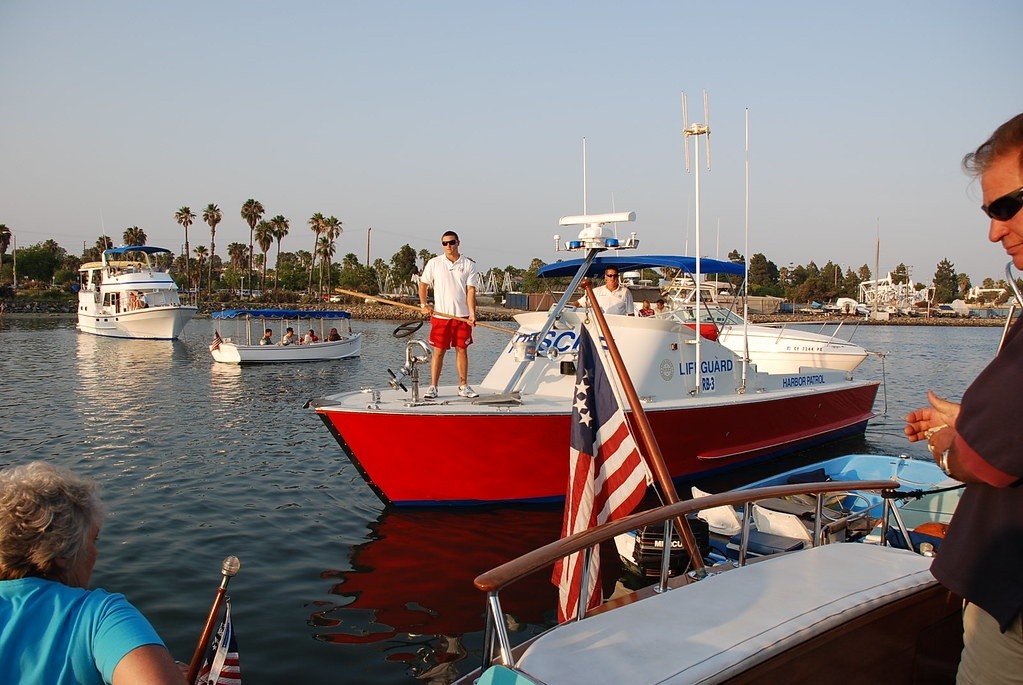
[231,288,262,299]
[323,294,347,304]
[299,291,308,296]
[933,304,957,318]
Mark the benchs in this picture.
[473,479,951,685]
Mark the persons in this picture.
[0,460,187,685]
[418,232,480,398]
[300,329,319,341]
[82,262,149,310]
[904,114,1023,685]
[638,299,654,318]
[654,299,669,314]
[282,327,294,346]
[324,327,342,343]
[260,329,274,345]
[570,265,634,316]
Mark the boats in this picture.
[208,307,365,365]
[615,449,967,599]
[76,241,197,340]
[452,272,955,685]
[296,88,897,511]
[616,233,859,323]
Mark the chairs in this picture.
[753,504,848,548]
[691,486,755,535]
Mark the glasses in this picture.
[606,273,618,278]
[442,240,455,246]
[982,187,1023,222]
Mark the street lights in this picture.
[2,230,17,289]
[241,276,244,300]
[366,227,372,268]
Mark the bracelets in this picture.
[940,448,950,475]
[420,303,428,309]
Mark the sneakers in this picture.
[424,386,438,397]
[458,385,479,397]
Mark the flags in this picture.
[211,331,221,353]
[200,598,242,685]
[552,319,654,626]
